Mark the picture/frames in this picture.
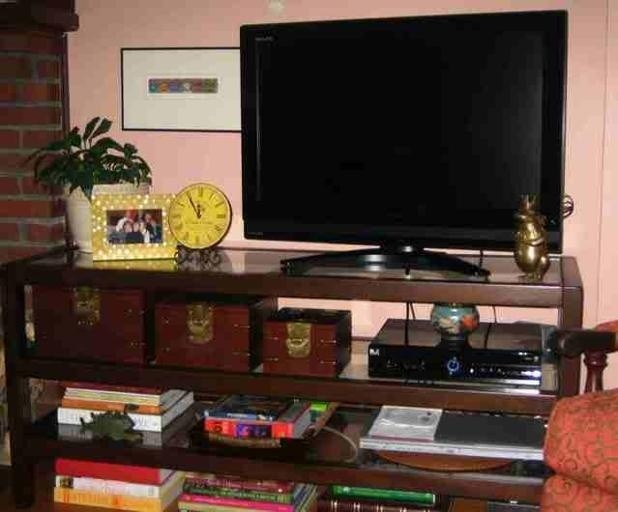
[91,192,178,261]
[116,46,242,134]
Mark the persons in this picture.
[111,210,159,243]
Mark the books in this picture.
[57,387,196,448]
[52,457,185,511]
[207,394,313,439]
[329,485,436,512]
[177,472,321,512]
[370,405,442,441]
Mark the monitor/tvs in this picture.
[240,9,568,276]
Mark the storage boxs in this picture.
[263,307,352,378]
[155,294,278,373]
[32,283,156,370]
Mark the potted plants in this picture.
[19,117,154,253]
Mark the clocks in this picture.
[167,183,231,264]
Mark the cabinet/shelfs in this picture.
[1,245,583,512]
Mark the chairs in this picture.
[540,322,618,512]
